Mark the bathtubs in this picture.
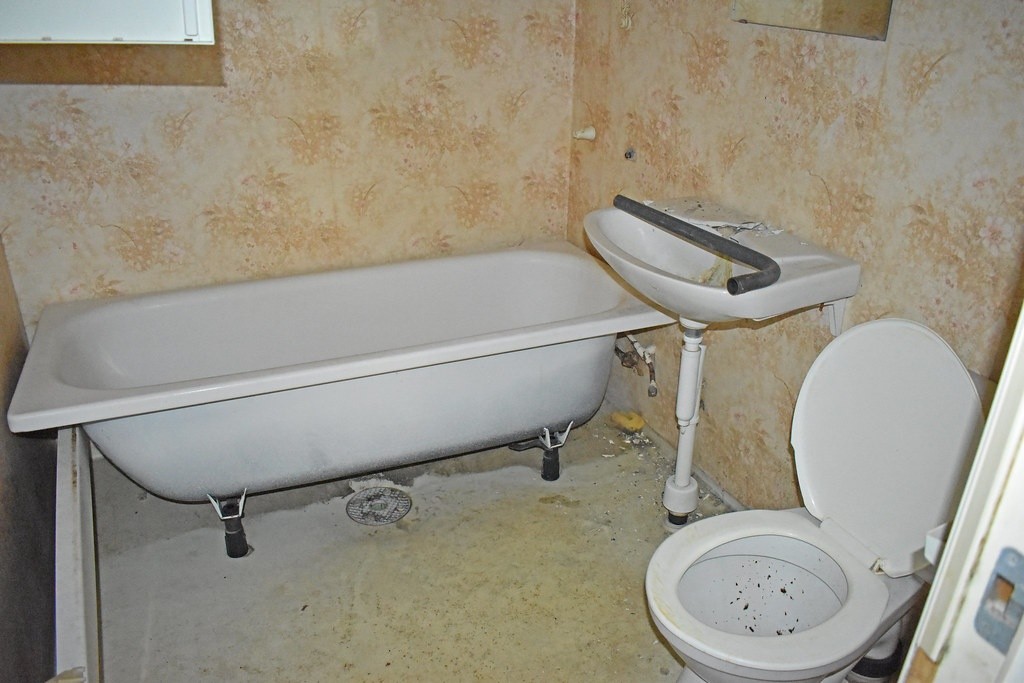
[6,236,683,504]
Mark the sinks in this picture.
[582,196,863,325]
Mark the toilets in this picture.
[643,313,1002,683]
